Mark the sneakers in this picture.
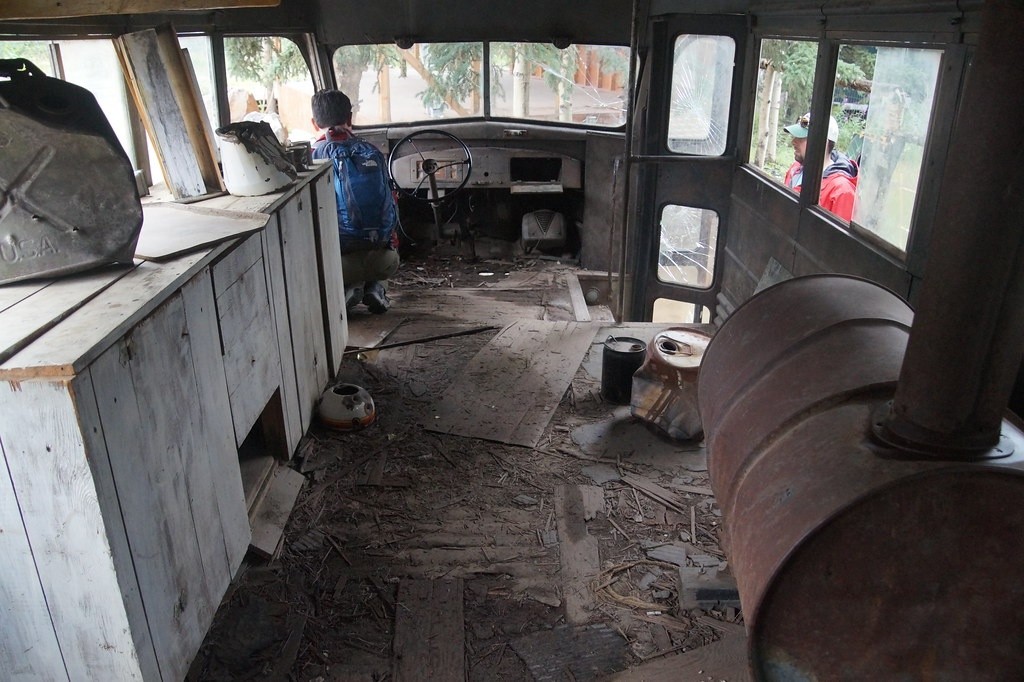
[362,281,391,312]
[345,281,364,312]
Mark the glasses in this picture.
[796,115,809,130]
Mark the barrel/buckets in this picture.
[601,335,648,406]
[696,272,1024,682]
[630,327,715,445]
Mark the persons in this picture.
[307,90,401,312]
[750,112,860,225]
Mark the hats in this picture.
[783,112,839,143]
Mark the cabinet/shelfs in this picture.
[0,158,350,682]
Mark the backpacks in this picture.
[311,126,399,243]
